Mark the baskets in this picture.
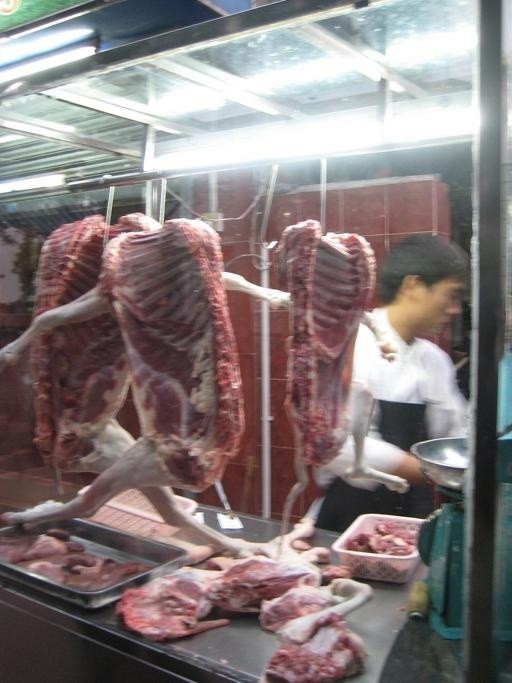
[332,512,426,585]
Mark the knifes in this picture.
[377,579,461,682]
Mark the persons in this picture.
[295,231,467,537]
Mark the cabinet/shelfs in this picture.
[0,1,510,682]
